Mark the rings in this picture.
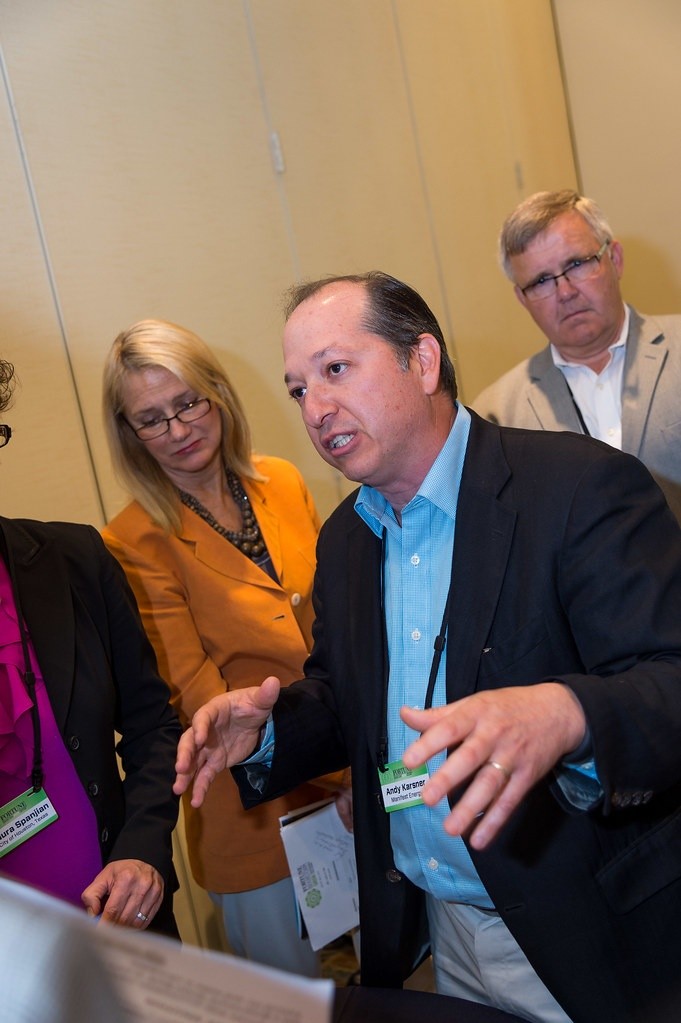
[137,912,147,922]
[486,761,510,778]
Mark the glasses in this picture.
[125,395,211,442]
[0,424,12,448]
[517,243,609,301]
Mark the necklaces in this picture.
[178,463,269,555]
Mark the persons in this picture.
[98,321,363,982]
[174,275,681,1021]
[465,189,681,525]
[0,356,187,943]
